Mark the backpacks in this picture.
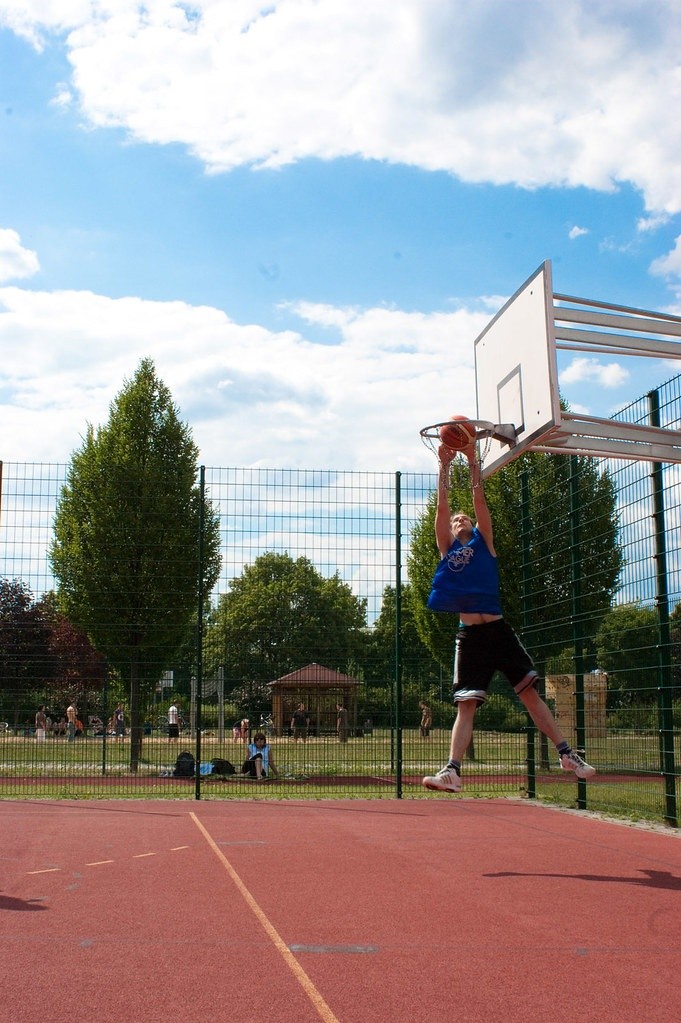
[173,750,195,776]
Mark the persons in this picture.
[336,703,350,743]
[241,733,278,780]
[36,704,46,741]
[420,442,596,792]
[419,701,432,736]
[233,718,249,744]
[25,714,117,735]
[291,703,310,744]
[114,701,126,743]
[167,700,180,743]
[66,701,79,742]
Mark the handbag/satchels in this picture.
[211,758,237,774]
[194,763,215,775]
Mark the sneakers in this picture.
[559,749,596,778]
[423,763,462,793]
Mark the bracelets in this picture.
[470,462,479,466]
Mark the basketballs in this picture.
[439,415,477,451]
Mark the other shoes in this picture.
[256,775,271,781]
